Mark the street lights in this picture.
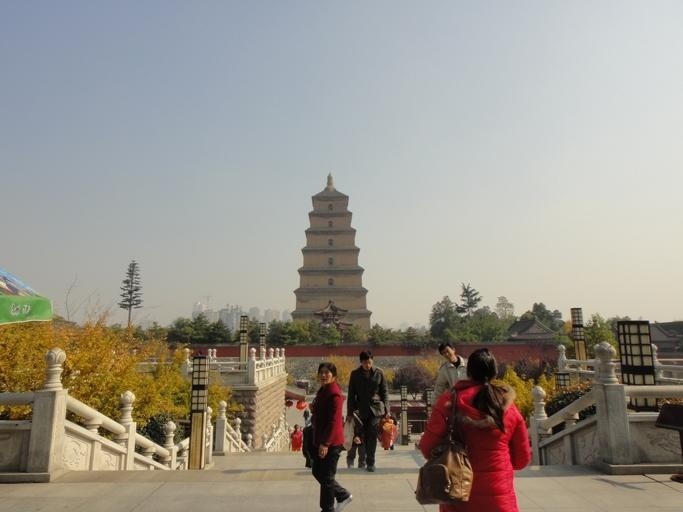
[400,386,409,444]
[424,389,435,423]
[259,322,267,360]
[188,356,209,469]
[554,372,570,394]
[617,320,659,412]
[571,307,588,370]
[240,316,249,362]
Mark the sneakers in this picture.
[335,495,352,512]
[367,465,375,471]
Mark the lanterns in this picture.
[285,400,294,408]
[296,400,307,410]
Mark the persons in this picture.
[288,424,303,452]
[430,342,471,405]
[416,347,533,511]
[382,412,397,449]
[303,410,312,426]
[345,350,392,472]
[303,415,315,469]
[346,428,365,469]
[311,362,354,512]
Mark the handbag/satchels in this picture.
[378,417,396,450]
[416,439,473,504]
